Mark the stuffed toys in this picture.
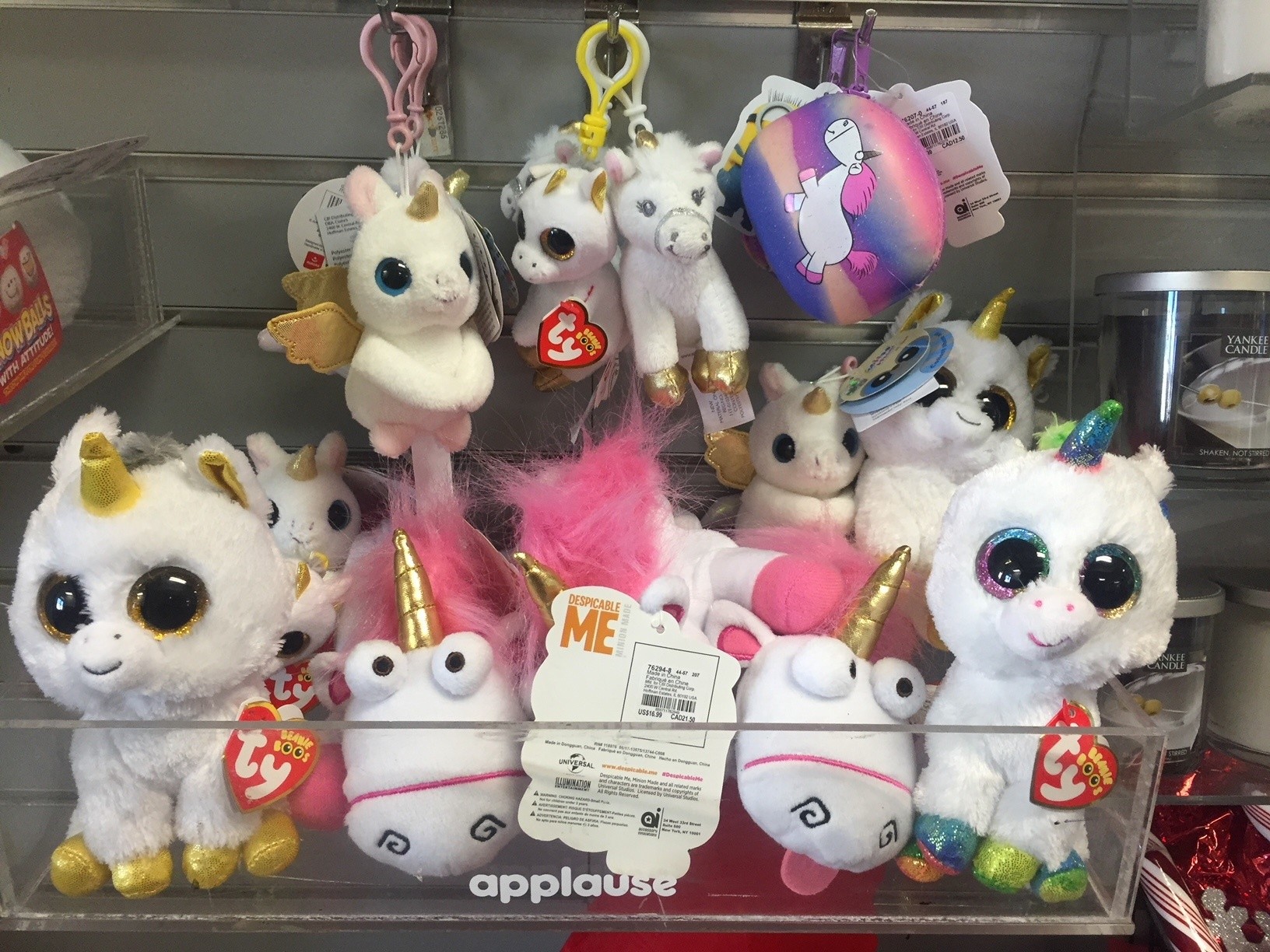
[8,117,1177,899]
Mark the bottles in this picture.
[1092,268,1270,480]
[1096,574,1225,775]
[1204,566,1270,767]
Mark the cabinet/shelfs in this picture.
[0,2,1270,934]
